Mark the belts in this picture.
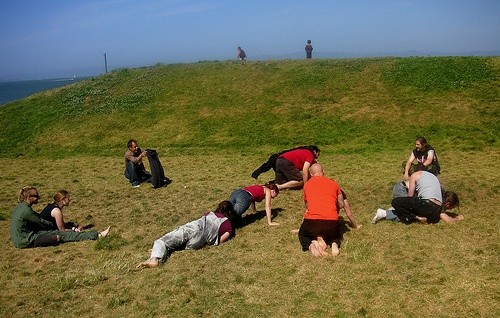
[401,181,409,192]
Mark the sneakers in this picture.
[131,180,140,188]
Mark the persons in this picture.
[290,188,363,236]
[298,164,345,258]
[390,164,443,226]
[11,186,111,249]
[305,39,313,59]
[123,139,151,186]
[136,201,235,267]
[237,47,247,61]
[251,145,291,179]
[40,190,83,233]
[228,182,281,226]
[267,145,322,192]
[402,135,441,179]
[372,178,465,225]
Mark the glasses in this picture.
[29,194,38,198]
